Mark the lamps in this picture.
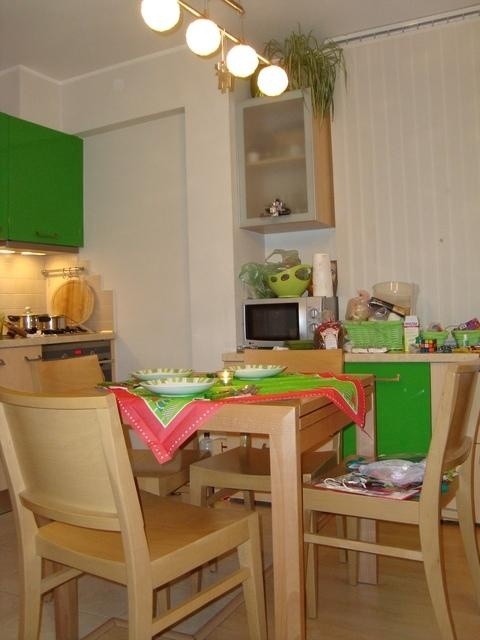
[139,0,292,98]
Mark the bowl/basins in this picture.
[129,368,192,382]
[139,377,221,397]
[226,363,289,378]
[372,281,411,307]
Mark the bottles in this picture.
[240,433,251,447]
[463,333,469,352]
[199,431,216,456]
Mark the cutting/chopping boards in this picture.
[50,280,97,326]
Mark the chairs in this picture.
[184,345,353,621]
[0,387,277,640]
[304,355,480,640]
[0,353,210,616]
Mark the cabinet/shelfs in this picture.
[342,354,480,525]
[0,112,84,257]
[238,88,333,235]
[0,338,43,492]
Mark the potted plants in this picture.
[252,24,349,124]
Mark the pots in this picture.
[7,305,70,336]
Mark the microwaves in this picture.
[239,295,339,350]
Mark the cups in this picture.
[246,144,303,162]
[204,369,218,378]
[215,369,234,387]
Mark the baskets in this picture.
[343,320,403,351]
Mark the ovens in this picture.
[40,341,112,383]
